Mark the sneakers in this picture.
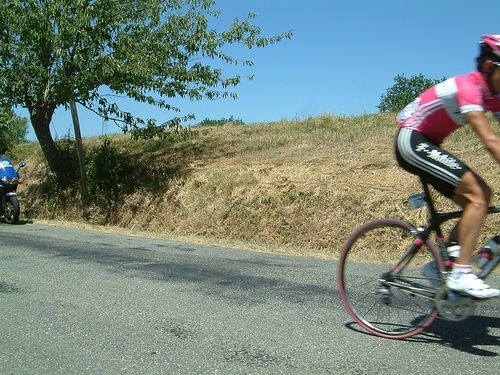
[422,261,452,321]
[446,264,499,298]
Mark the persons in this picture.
[0,157,19,217]
[392,33,500,301]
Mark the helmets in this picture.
[476,34,500,80]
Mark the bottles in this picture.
[469,235,500,274]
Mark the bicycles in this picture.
[337,175,500,340]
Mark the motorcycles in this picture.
[0,156,26,223]
